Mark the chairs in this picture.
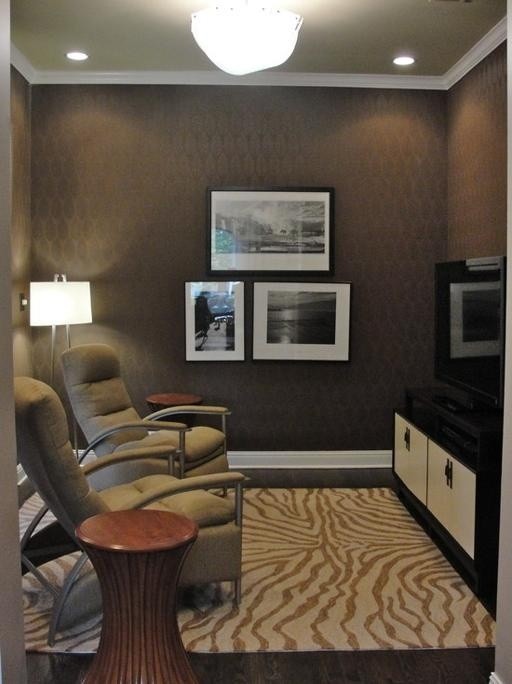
[59,343,232,497]
[13,375,251,647]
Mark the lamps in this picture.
[30,274,93,460]
[190,5,302,78]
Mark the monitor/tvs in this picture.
[433,254,506,416]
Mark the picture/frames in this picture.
[205,186,334,276]
[251,280,354,365]
[450,282,503,359]
[184,280,245,364]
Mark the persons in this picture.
[195,292,214,351]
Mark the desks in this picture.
[146,392,203,428]
[75,508,200,684]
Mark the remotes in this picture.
[433,395,466,414]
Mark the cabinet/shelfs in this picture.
[392,385,503,621]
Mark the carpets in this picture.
[17,487,495,653]
